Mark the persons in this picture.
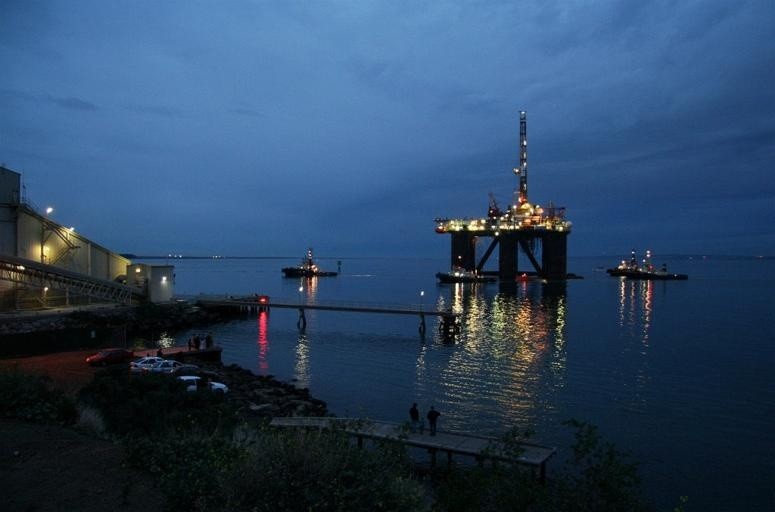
[193,335,201,349]
[206,331,213,350]
[427,405,441,437]
[188,339,192,350]
[157,348,164,357]
[408,402,419,434]
[419,417,426,435]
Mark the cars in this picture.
[86,348,134,368]
[128,356,229,396]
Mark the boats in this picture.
[281,249,338,278]
[436,272,496,284]
[606,248,688,281]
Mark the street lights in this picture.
[41,207,53,265]
